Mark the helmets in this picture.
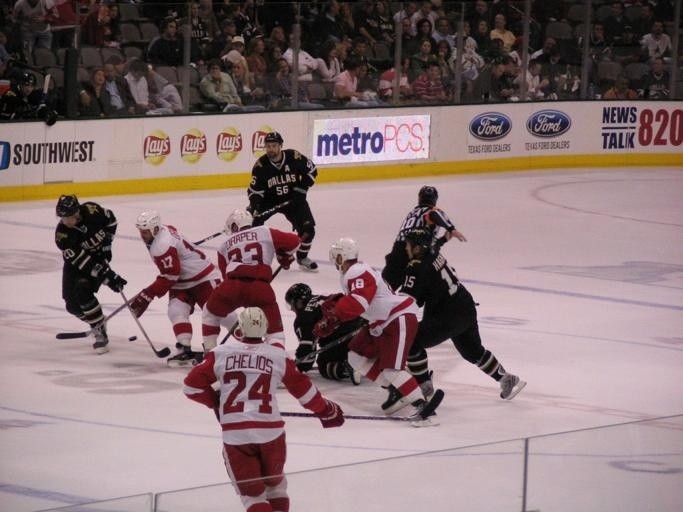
[266,132,283,143]
[21,72,37,87]
[285,284,311,311]
[238,307,268,338]
[224,209,253,236]
[408,226,432,249]
[420,186,438,203]
[329,238,358,264]
[56,194,79,224]
[136,209,159,241]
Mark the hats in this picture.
[220,18,237,30]
[232,36,245,45]
[623,25,633,33]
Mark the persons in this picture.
[285,282,370,385]
[398,226,520,427]
[131,209,242,355]
[200,207,301,351]
[378,184,467,295]
[0,0,683,124]
[311,236,430,420]
[52,193,129,355]
[244,131,320,272]
[182,306,345,512]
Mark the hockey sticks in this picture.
[280,389,444,422]
[102,257,171,358]
[168,264,281,365]
[56,289,145,339]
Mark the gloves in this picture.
[252,204,265,227]
[21,109,37,120]
[131,288,155,318]
[98,264,127,292]
[321,293,345,314]
[290,180,308,208]
[314,400,345,428]
[276,253,295,270]
[313,311,344,337]
[43,110,57,125]
[99,236,113,264]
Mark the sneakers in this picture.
[499,373,519,399]
[93,334,108,349]
[420,369,434,402]
[296,256,318,269]
[344,358,361,386]
[409,401,436,421]
[186,350,203,364]
[167,350,195,362]
[381,383,404,410]
